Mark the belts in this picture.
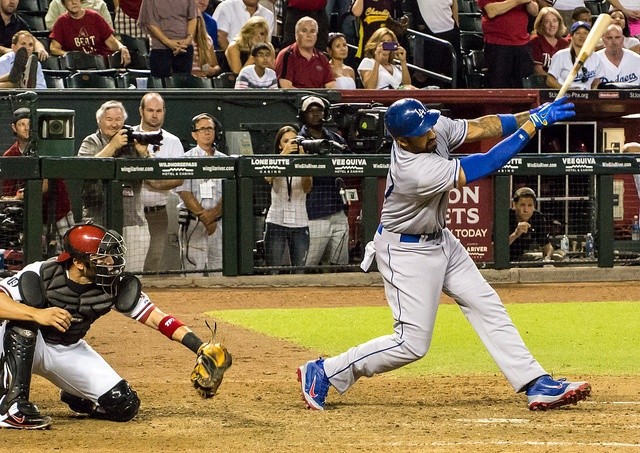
[375,224,442,242]
[144,205,165,213]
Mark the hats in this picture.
[571,20,591,31]
[12,107,30,136]
[302,97,325,112]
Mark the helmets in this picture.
[384,98,441,139]
[511,187,537,210]
[55,221,128,298]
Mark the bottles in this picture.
[585,233,594,259]
[632,215,639,239]
[560,234,570,254]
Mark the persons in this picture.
[45,0,131,68]
[476,1,539,88]
[132,92,185,271]
[234,44,280,89]
[0,30,47,87]
[412,0,466,87]
[284,2,329,50]
[357,29,411,90]
[584,0,640,20]
[0,0,49,60]
[622,142,640,195]
[211,0,280,48]
[261,125,312,273]
[274,17,337,91]
[115,0,224,82]
[0,223,232,431]
[296,98,591,412]
[571,6,594,28]
[76,101,150,276]
[326,33,357,92]
[349,0,409,66]
[597,8,639,53]
[176,113,228,277]
[219,16,277,77]
[297,94,353,268]
[1,107,68,223]
[546,20,604,90]
[596,24,639,94]
[509,187,554,261]
[524,7,570,76]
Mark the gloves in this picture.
[528,95,575,130]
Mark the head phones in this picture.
[514,191,542,211]
[191,112,222,133]
[296,95,331,122]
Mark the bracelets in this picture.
[186,33,195,40]
[497,114,517,136]
[158,315,187,338]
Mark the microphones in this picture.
[320,115,332,122]
[211,135,224,146]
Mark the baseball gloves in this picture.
[190,320,232,399]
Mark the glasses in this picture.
[256,53,270,57]
[194,127,215,134]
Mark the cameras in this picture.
[383,41,398,50]
[122,123,163,157]
[328,103,385,155]
[37,106,75,155]
[296,135,329,154]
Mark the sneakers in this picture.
[20,55,38,88]
[295,357,332,410]
[526,375,591,410]
[9,47,28,82]
[0,394,54,430]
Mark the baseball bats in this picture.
[553,13,613,102]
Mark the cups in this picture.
[136,77,149,89]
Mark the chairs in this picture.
[15,0,363,89]
[399,0,602,90]
